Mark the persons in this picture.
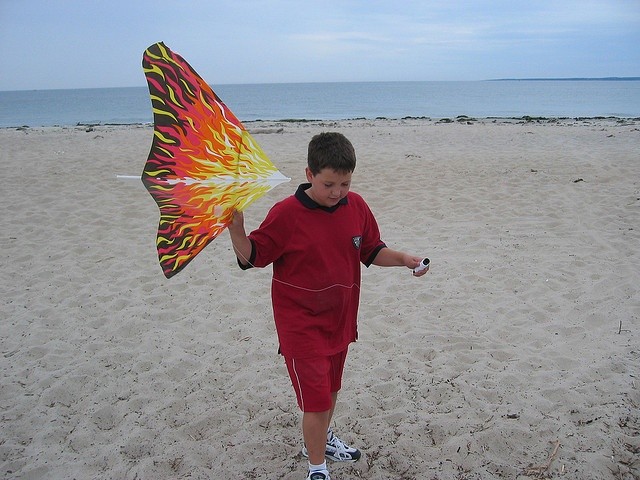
[225,132,430,480]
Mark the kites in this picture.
[115,40,291,280]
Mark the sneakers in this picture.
[306,470,331,480]
[301,429,361,463]
